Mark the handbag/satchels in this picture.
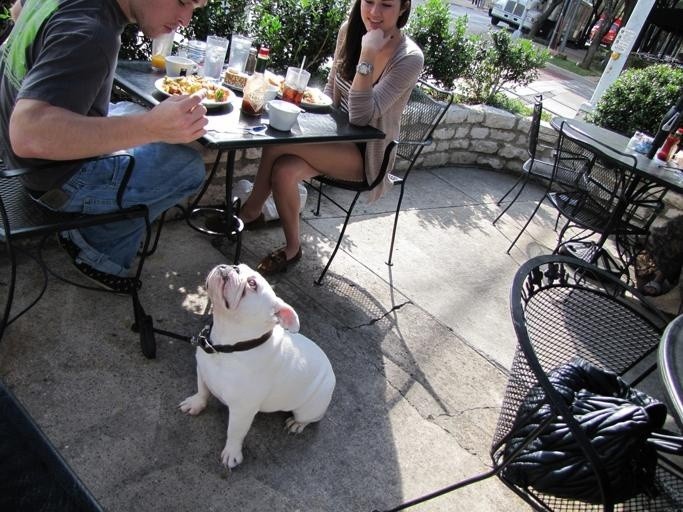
[502,359,667,505]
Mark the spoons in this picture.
[206,124,267,134]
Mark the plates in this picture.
[220,81,243,93]
[276,85,333,109]
[154,75,234,109]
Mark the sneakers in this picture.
[57,234,142,296]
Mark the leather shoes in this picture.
[206,212,264,232]
[256,246,302,273]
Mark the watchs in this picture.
[356,62,374,76]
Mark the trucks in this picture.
[586,14,621,48]
[487,0,593,48]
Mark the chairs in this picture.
[505,117,672,285]
[491,92,594,228]
[301,72,457,284]
[382,250,682,508]
[0,135,151,348]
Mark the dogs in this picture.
[177,260,336,469]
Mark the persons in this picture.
[203,1,425,279]
[635,216,683,296]
[0,0,209,297]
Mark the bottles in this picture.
[244,48,258,76]
[255,47,270,75]
[657,128,683,162]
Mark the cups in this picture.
[228,35,254,73]
[204,34,229,78]
[150,29,174,71]
[242,80,265,116]
[165,55,197,76]
[268,100,300,133]
[282,66,311,105]
[263,83,277,101]
